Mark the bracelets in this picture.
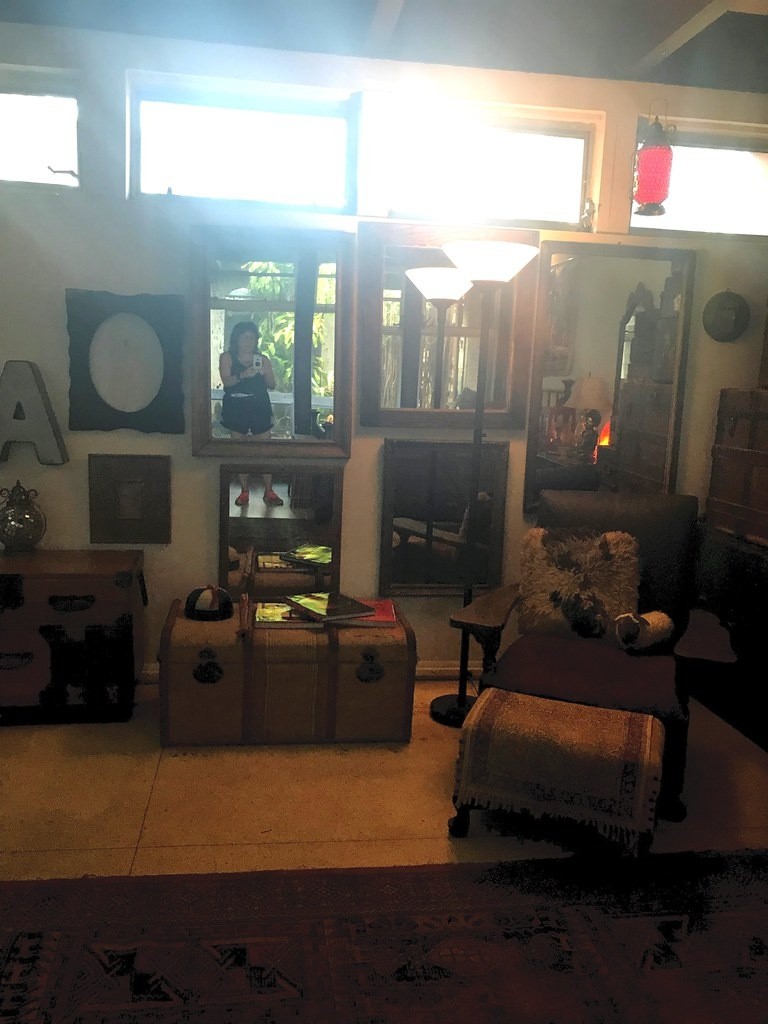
[237,374,243,383]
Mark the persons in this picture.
[219,321,283,507]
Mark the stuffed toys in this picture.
[614,610,674,653]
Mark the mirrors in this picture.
[522,240,699,498]
[182,231,354,460]
[357,220,538,432]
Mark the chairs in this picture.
[448,488,737,880]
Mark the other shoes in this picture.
[264,492,283,505]
[235,491,249,504]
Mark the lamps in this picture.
[440,232,542,609]
[404,267,473,585]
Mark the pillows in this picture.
[517,525,640,645]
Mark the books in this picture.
[254,591,397,629]
[257,543,333,574]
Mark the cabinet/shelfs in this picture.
[0,550,147,728]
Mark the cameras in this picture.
[252,354,263,374]
